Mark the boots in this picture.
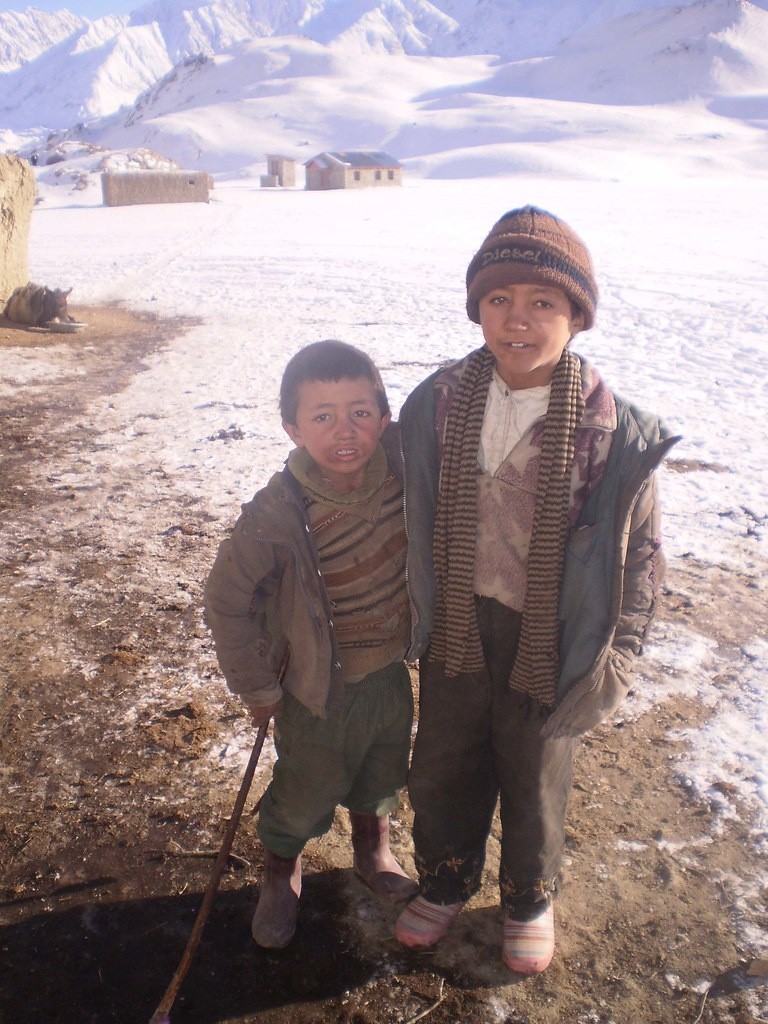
[252,847,302,948]
[349,808,419,902]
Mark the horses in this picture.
[1,285,84,326]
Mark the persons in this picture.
[393,202,682,970]
[200,338,423,947]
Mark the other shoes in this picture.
[503,898,555,975]
[395,894,469,949]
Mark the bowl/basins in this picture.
[47,323,86,333]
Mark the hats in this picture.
[467,205,598,329]
